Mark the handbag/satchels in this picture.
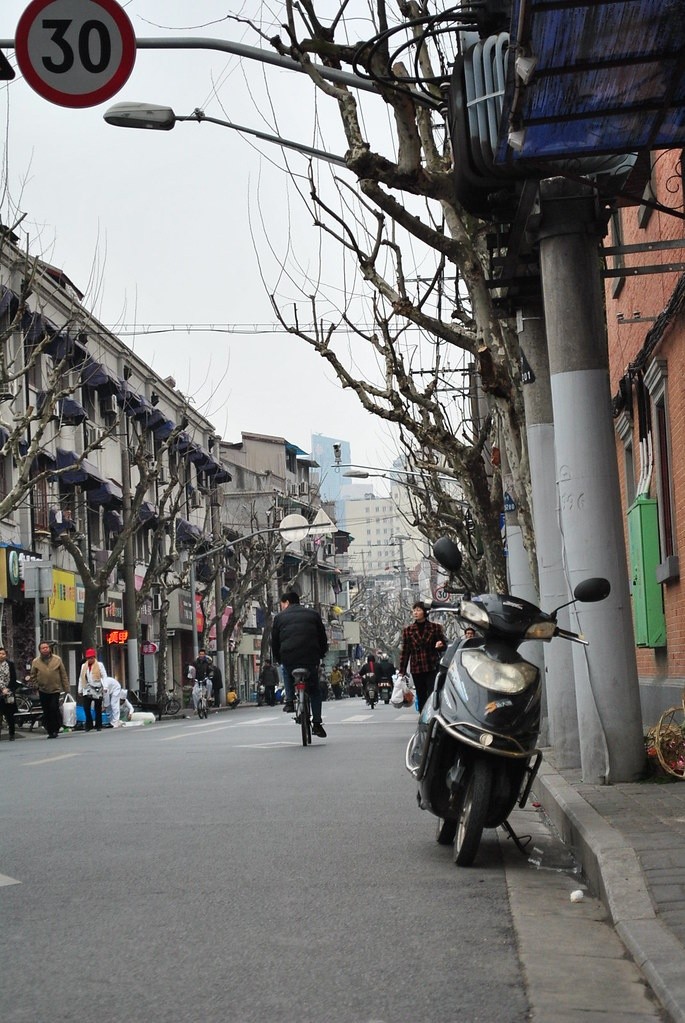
[62,694,77,728]
[391,675,413,709]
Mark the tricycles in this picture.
[131,678,164,721]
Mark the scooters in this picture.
[405,537,612,868]
[15,676,41,707]
[362,673,379,709]
[378,676,393,705]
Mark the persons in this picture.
[31,643,71,739]
[317,662,363,702]
[99,674,134,727]
[0,648,19,742]
[260,658,286,707]
[379,654,396,683]
[399,602,448,716]
[357,656,383,699]
[465,627,475,637]
[227,687,239,708]
[272,591,329,738]
[78,648,109,732]
[188,650,214,713]
[211,665,223,708]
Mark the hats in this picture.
[119,689,127,699]
[86,649,96,659]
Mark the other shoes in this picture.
[85,725,94,732]
[105,723,113,728]
[97,727,102,732]
[282,701,295,713]
[207,696,213,701]
[47,732,58,739]
[194,709,198,715]
[9,734,15,741]
[312,724,327,738]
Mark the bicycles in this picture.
[278,654,326,747]
[1,695,32,724]
[188,676,214,720]
[162,688,181,715]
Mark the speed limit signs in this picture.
[14,0,137,110]
[435,586,451,602]
